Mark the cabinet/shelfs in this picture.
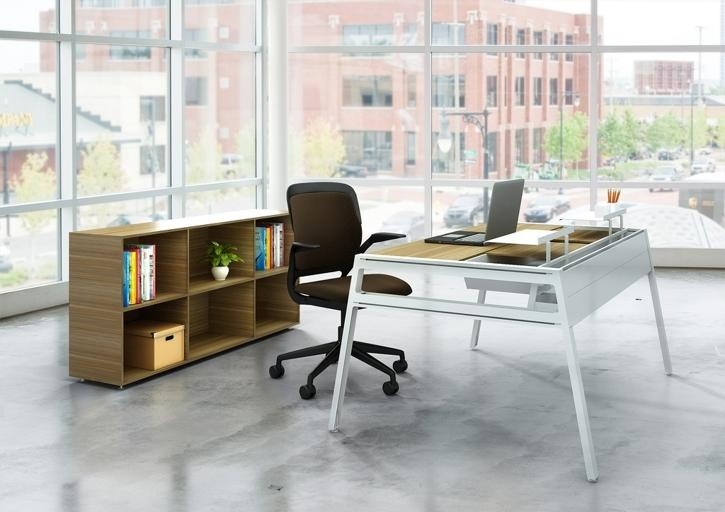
[68,209,299,390]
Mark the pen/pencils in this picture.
[608,187,620,203]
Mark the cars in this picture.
[656,145,682,160]
[646,159,716,192]
[338,158,378,177]
[364,143,392,161]
[0,237,13,271]
[523,195,570,221]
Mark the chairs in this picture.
[270,182,415,399]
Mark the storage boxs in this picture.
[124,321,185,371]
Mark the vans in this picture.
[221,153,243,181]
[444,191,491,227]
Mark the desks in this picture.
[328,221,672,482]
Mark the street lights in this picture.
[438,106,491,224]
[558,93,581,194]
[0,135,13,238]
[691,93,706,163]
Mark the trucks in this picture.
[513,164,539,193]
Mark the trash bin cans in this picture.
[679,186,725,221]
[222,154,239,181]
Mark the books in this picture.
[255,220,285,271]
[123,242,158,309]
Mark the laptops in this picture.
[424,177,525,247]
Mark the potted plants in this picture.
[207,240,245,280]
